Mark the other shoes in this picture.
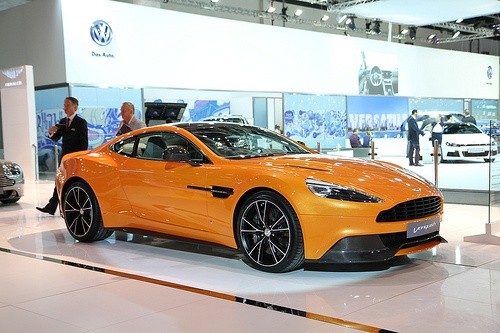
[409,163,424,166]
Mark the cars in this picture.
[418,121,499,163]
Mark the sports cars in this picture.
[54,121,448,274]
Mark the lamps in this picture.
[373,18,382,35]
[408,26,417,40]
[346,15,357,31]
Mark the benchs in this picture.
[311,147,376,156]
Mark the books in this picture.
[115,124,133,137]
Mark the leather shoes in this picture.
[36,207,54,215]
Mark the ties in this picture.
[66,118,69,128]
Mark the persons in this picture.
[362,132,372,148]
[409,110,426,166]
[350,127,362,148]
[109,102,151,156]
[463,109,477,125]
[36,97,89,216]
[430,113,442,156]
